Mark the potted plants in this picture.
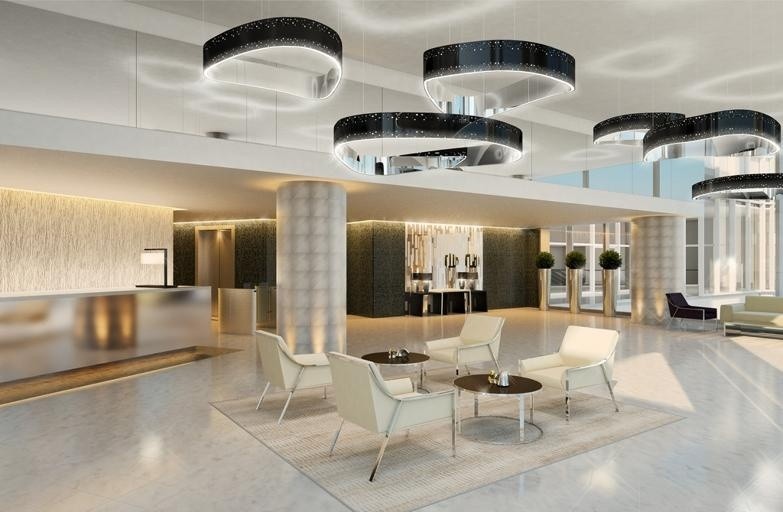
[598,249,623,316]
[563,250,587,313]
[534,252,556,312]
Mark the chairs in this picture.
[251,326,331,426]
[424,314,505,373]
[326,348,457,483]
[518,324,621,420]
[664,291,718,333]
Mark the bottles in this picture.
[496,369,510,387]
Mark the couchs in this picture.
[720,296,782,339]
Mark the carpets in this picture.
[208,364,688,512]
